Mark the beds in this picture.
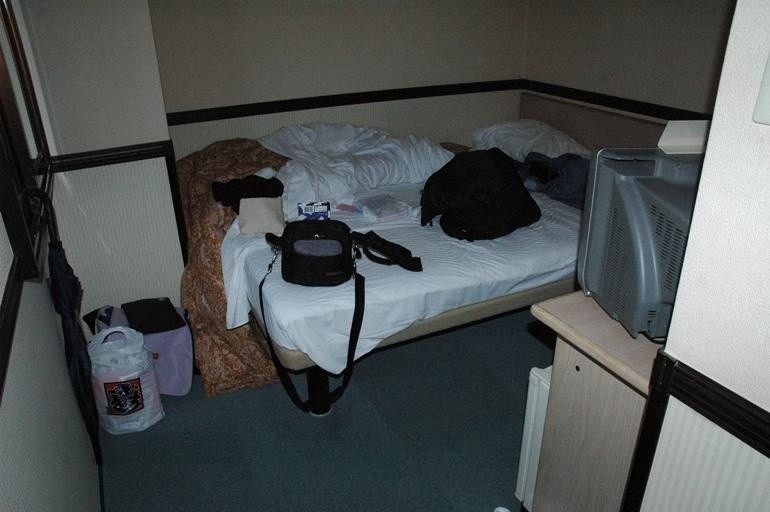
[176,94,593,419]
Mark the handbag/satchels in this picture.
[82,304,193,436]
[279,218,356,287]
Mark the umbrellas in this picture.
[17,184,103,466]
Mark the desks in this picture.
[516,287,674,512]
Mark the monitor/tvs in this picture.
[576,147,707,344]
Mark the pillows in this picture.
[472,115,590,167]
